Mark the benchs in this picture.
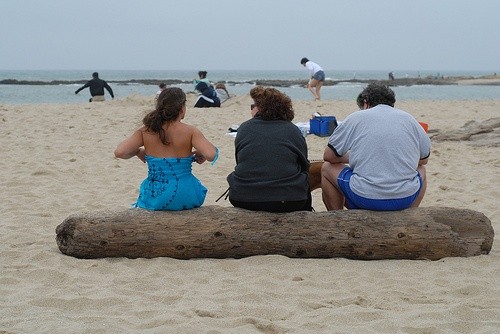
[56,205,495,261]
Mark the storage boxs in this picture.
[310,116,338,137]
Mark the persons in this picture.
[190,70,210,94]
[193,83,230,108]
[226,86,316,213]
[321,83,431,211]
[155,83,166,94]
[301,57,325,101]
[75,72,114,102]
[114,87,218,211]
[388,71,395,80]
